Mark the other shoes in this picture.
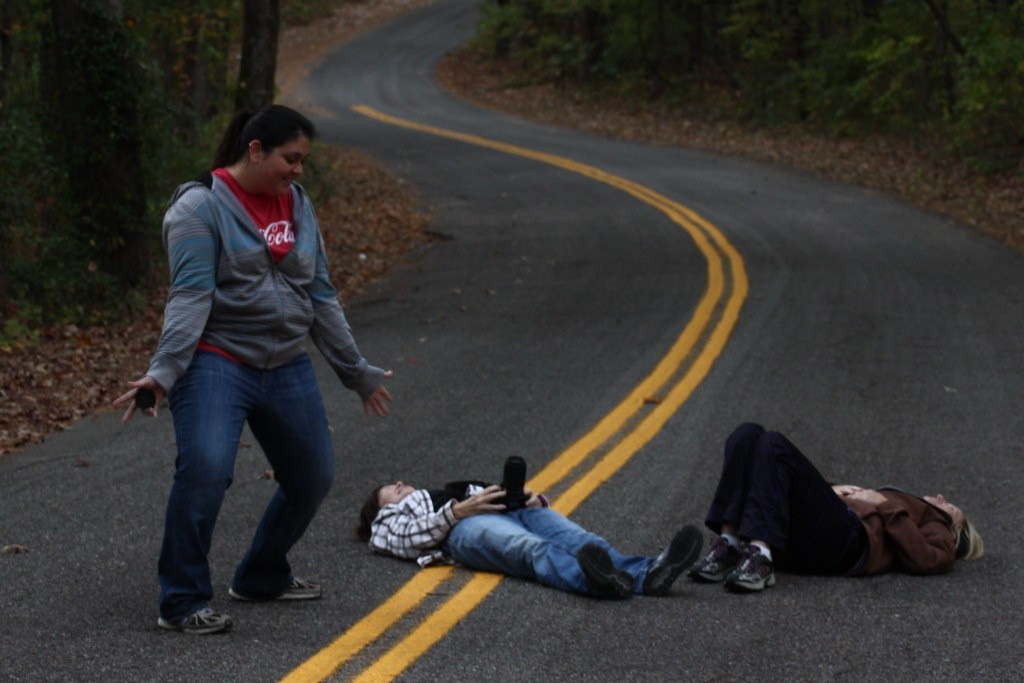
[577,543,635,600]
[642,526,703,595]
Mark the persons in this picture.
[352,482,705,600]
[109,106,396,635]
[687,423,984,591]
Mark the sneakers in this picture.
[724,545,776,591]
[698,536,743,581]
[228,571,322,599]
[158,607,232,635]
